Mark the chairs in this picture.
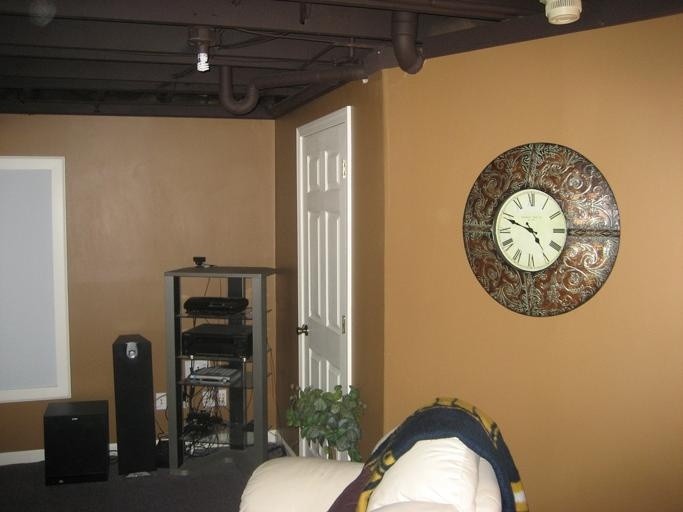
[239,397,502,512]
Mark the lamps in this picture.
[188,29,222,73]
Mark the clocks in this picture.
[462,143,620,317]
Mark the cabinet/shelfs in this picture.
[165,265,275,468]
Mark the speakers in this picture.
[150,438,184,469]
[112,333,157,474]
[44,400,110,487]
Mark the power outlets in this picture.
[155,392,167,410]
[183,357,225,408]
